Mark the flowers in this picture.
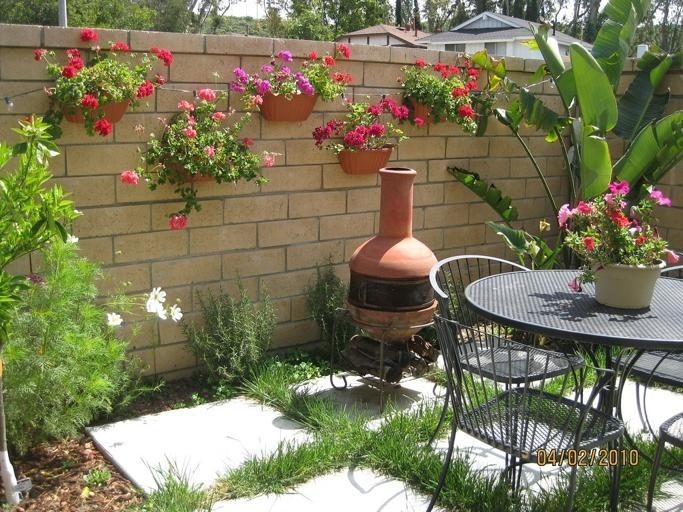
[229,42,352,121]
[313,95,424,154]
[119,87,276,227]
[396,58,479,134]
[34,27,174,139]
[555,177,679,294]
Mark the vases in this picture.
[555,177,679,294]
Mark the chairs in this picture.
[425,255,683,512]
